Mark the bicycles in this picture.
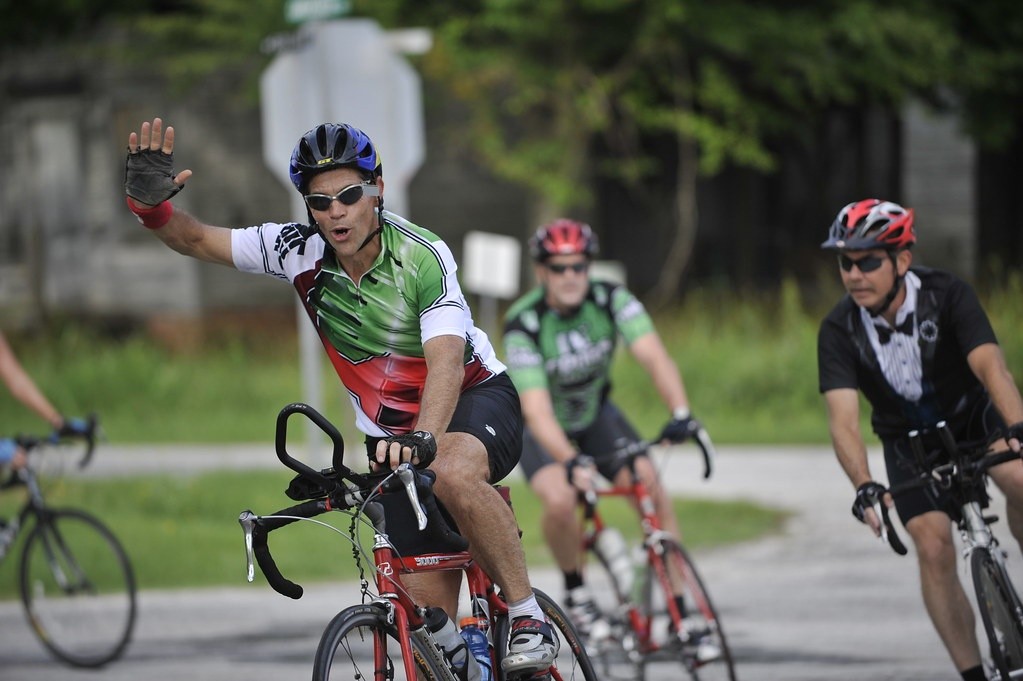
[239,402,601,681]
[1,414,139,668]
[561,417,736,681]
[877,421,1023,681]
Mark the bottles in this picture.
[596,528,637,596]
[423,606,482,681]
[458,616,494,681]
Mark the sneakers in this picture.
[667,611,719,660]
[565,584,605,637]
[501,615,561,674]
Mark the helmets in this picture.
[826,200,916,250]
[526,218,601,262]
[289,122,383,196]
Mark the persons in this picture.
[124,118,561,681]
[501,219,723,671]
[0,331,90,467]
[817,199,1022,681]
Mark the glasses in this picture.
[541,260,590,271]
[304,179,371,211]
[837,245,908,273]
